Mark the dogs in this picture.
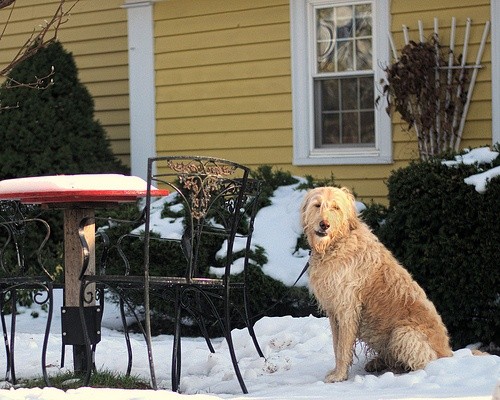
[301,186,454,383]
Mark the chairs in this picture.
[0,156,266,395]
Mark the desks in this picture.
[0,174,169,379]
